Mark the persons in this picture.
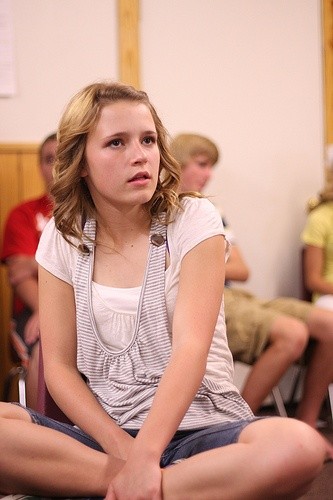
[0,77,328,500]
[1,130,69,428]
[300,164,333,327]
[158,131,333,463]
